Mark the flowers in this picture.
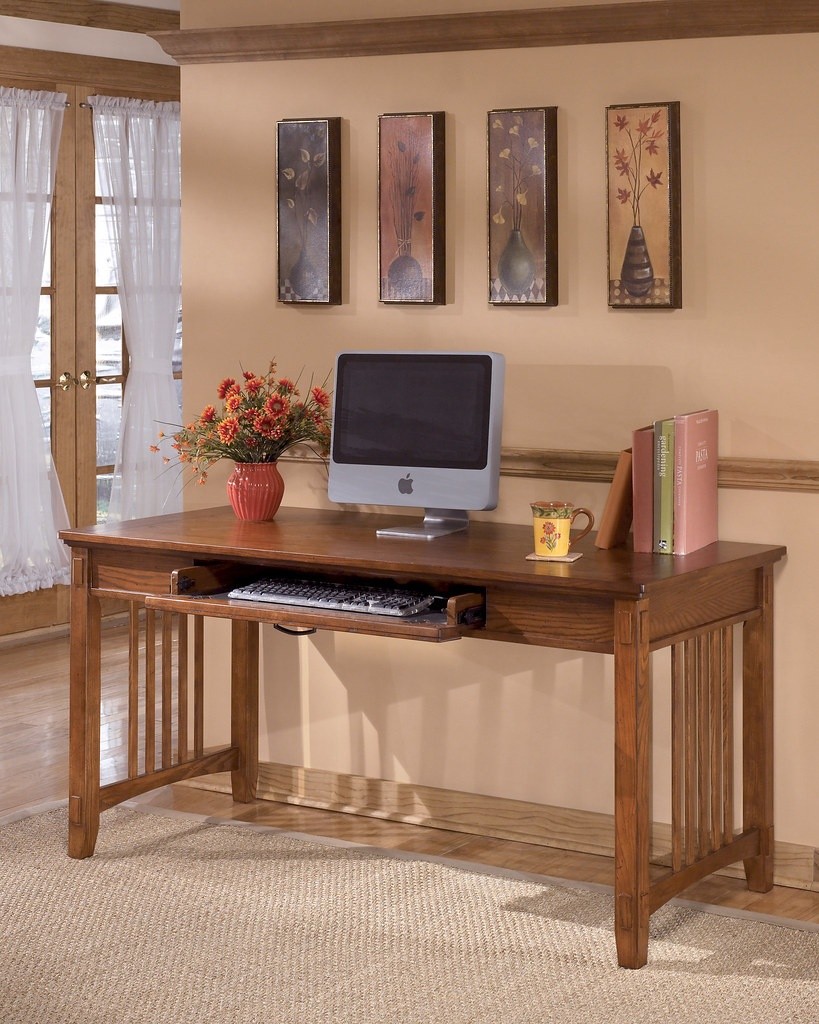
[152,356,331,492]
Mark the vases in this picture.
[226,460,285,522]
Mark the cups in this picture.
[530,501,594,556]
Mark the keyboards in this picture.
[228,576,435,618]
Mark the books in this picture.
[594,410,719,555]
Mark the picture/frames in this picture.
[605,98,683,311]
[376,111,443,307]
[487,107,557,306]
[273,118,341,305]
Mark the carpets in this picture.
[0,798,819,1024]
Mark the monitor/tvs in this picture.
[329,353,504,539]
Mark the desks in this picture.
[58,504,789,965]
